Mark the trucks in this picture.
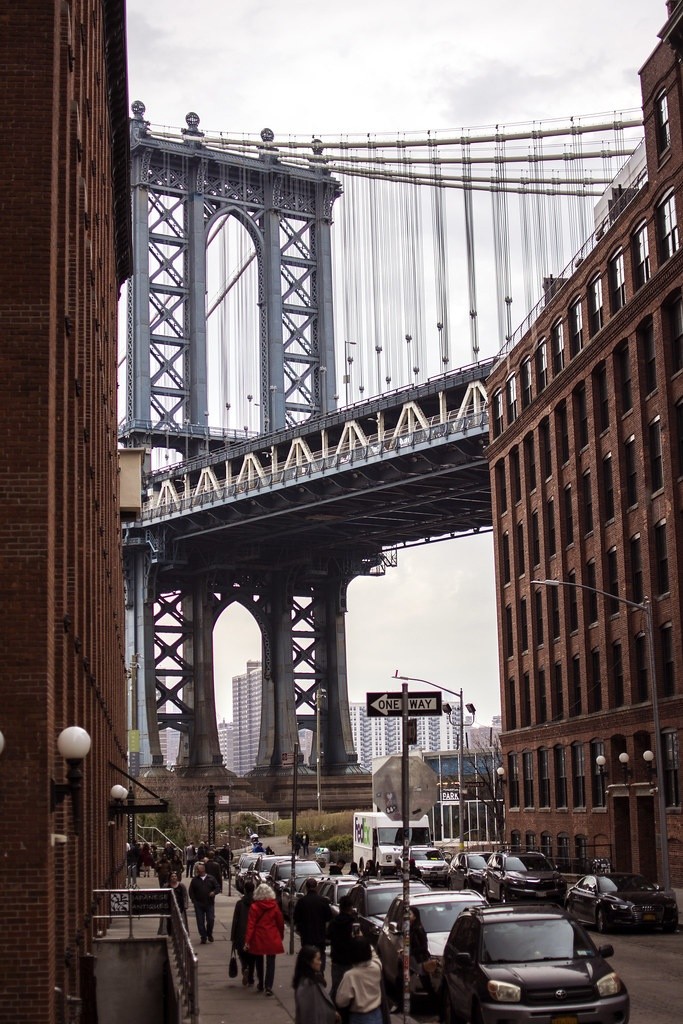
[351,812,433,879]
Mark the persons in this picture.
[328,860,346,874]
[348,862,360,877]
[326,896,362,1002]
[334,941,384,1024]
[409,858,423,880]
[363,860,375,877]
[293,877,331,988]
[292,946,341,1024]
[126,828,308,996]
[388,907,431,1014]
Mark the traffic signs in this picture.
[368,692,444,719]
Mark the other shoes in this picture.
[247,980,254,987]
[201,936,206,944]
[390,1004,404,1014]
[242,969,249,985]
[207,933,214,942]
[257,984,263,991]
[266,989,273,995]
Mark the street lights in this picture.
[531,577,672,894]
[390,675,477,855]
[316,684,327,816]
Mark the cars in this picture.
[446,851,493,891]
[396,845,450,884]
[375,890,495,1000]
[561,873,679,934]
[481,849,564,905]
[437,904,630,1024]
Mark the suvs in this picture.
[233,852,301,894]
[265,858,358,939]
[344,879,431,958]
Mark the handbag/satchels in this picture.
[228,946,238,978]
